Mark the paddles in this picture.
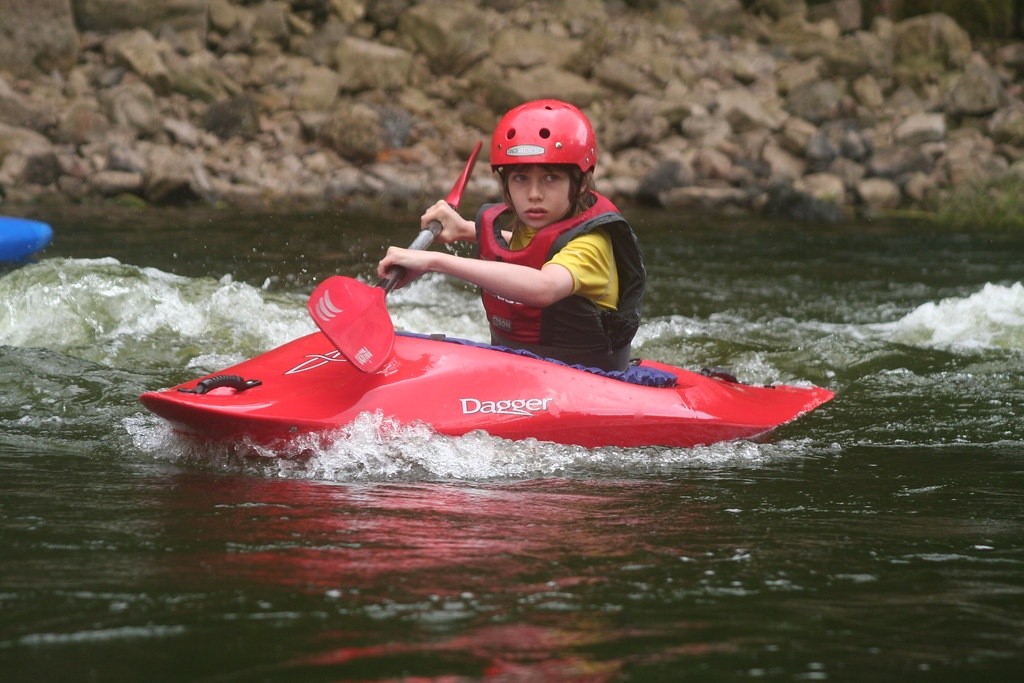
[306,139,483,375]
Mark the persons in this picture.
[378,99,646,373]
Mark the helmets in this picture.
[490,99,597,173]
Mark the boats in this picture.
[137,323,839,452]
